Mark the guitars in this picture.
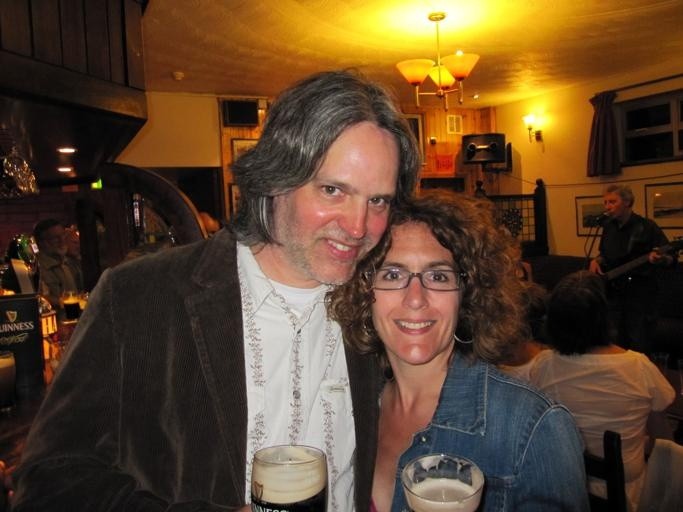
[605,234,683,281]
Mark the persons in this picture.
[33,219,85,297]
[7,68,424,511]
[587,182,678,357]
[169,199,223,246]
[524,268,678,511]
[323,190,590,511]
[493,279,552,385]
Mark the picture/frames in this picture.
[575,196,609,237]
[231,138,258,163]
[645,182,683,229]
[228,182,241,217]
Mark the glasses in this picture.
[362,268,467,291]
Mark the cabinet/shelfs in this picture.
[423,106,483,178]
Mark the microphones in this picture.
[596,212,610,222]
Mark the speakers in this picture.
[463,133,505,163]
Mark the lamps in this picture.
[396,12,480,113]
[525,115,542,143]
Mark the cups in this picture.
[402,452,484,512]
[250,444,330,512]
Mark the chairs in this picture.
[581,431,629,512]
